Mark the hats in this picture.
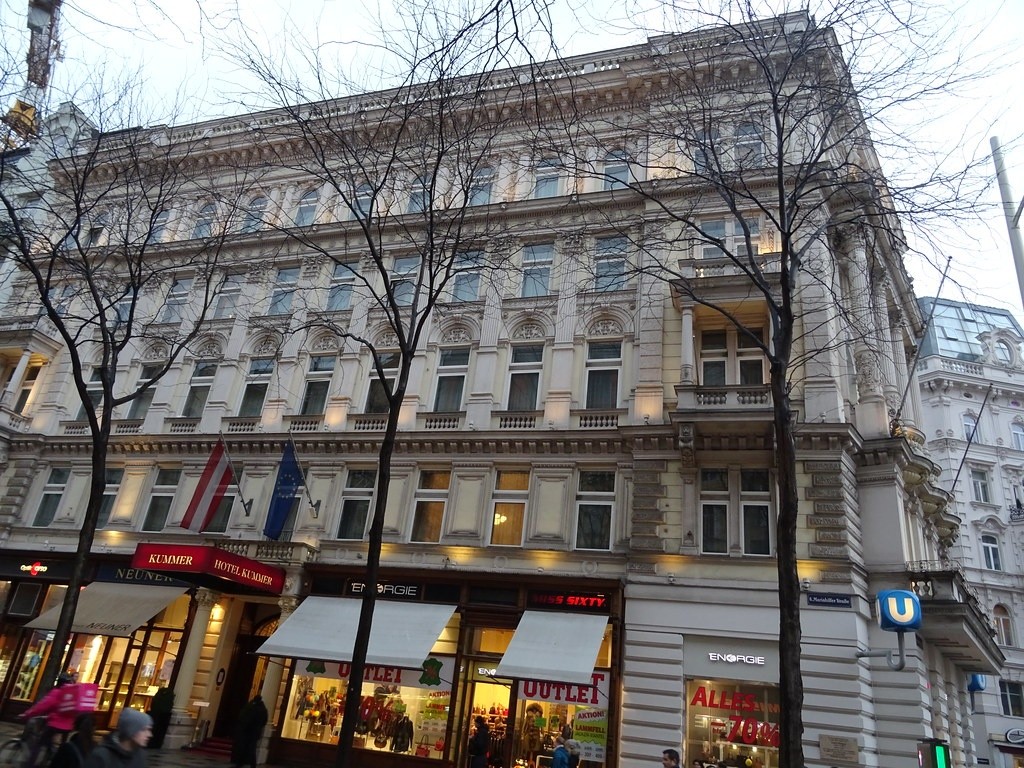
[117,707,153,743]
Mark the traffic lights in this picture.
[916,737,952,768]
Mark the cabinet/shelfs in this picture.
[469,713,508,742]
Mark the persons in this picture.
[0,672,704,768]
[520,703,545,751]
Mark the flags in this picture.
[181,434,233,534]
[263,439,302,539]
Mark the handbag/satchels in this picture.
[435,736,445,751]
[725,753,736,767]
[737,747,752,768]
[374,730,386,748]
[330,730,339,744]
[754,757,762,768]
[416,734,430,756]
[352,729,364,748]
[307,721,319,740]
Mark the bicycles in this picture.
[0,713,87,768]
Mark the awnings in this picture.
[254,595,459,668]
[492,611,608,685]
[22,580,189,637]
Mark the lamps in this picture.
[324,423,331,432]
[548,419,555,430]
[803,578,810,591]
[138,426,144,433]
[451,562,456,569]
[469,421,475,430]
[101,543,106,550]
[44,540,49,548]
[259,424,266,433]
[25,425,29,432]
[668,572,675,582]
[537,567,544,575]
[819,411,826,423]
[643,414,650,425]
